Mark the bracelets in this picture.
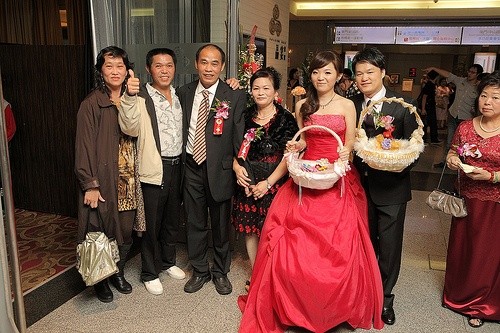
[489,171,498,184]
[266,179,271,189]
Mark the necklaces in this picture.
[318,93,336,109]
[256,109,275,118]
[480,114,500,133]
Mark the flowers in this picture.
[244,126,266,142]
[211,96,232,121]
[291,86,306,96]
[240,26,285,109]
[447,140,482,161]
[371,110,396,132]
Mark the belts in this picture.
[161,157,183,165]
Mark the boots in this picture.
[109,240,132,294]
[93,278,113,302]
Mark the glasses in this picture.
[465,68,477,75]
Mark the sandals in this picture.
[467,317,484,328]
[245,279,250,292]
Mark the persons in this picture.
[0,98,16,217]
[233,65,297,292]
[417,70,440,143]
[287,69,300,88]
[177,44,245,295]
[443,70,500,329]
[335,69,352,97]
[116,49,241,295]
[339,73,364,127]
[351,49,419,326]
[427,64,482,149]
[236,49,384,333]
[75,46,146,303]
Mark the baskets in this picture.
[354,97,424,172]
[283,125,351,190]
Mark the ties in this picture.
[192,90,211,165]
[365,100,375,137]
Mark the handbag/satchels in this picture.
[75,206,120,269]
[78,206,119,287]
[424,154,468,217]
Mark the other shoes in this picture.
[144,277,164,295]
[433,162,447,169]
[161,265,186,280]
[431,137,441,144]
[424,139,428,143]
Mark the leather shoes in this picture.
[213,277,232,295]
[184,274,211,293]
[382,306,395,325]
